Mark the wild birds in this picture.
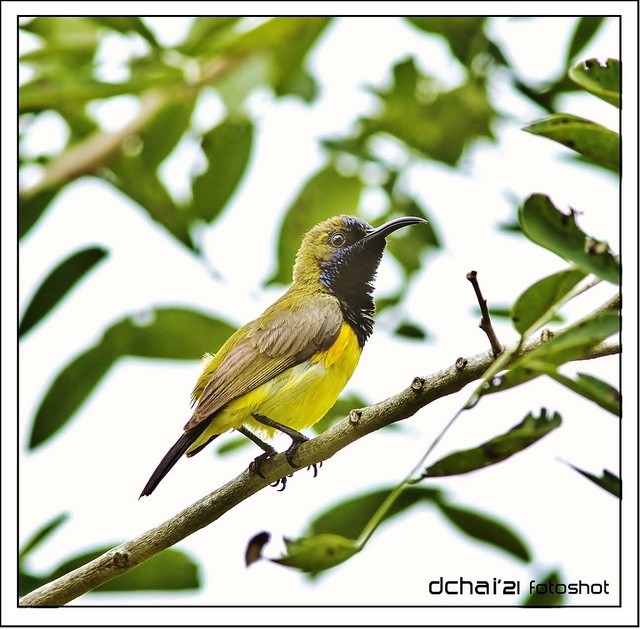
[136,213,430,501]
